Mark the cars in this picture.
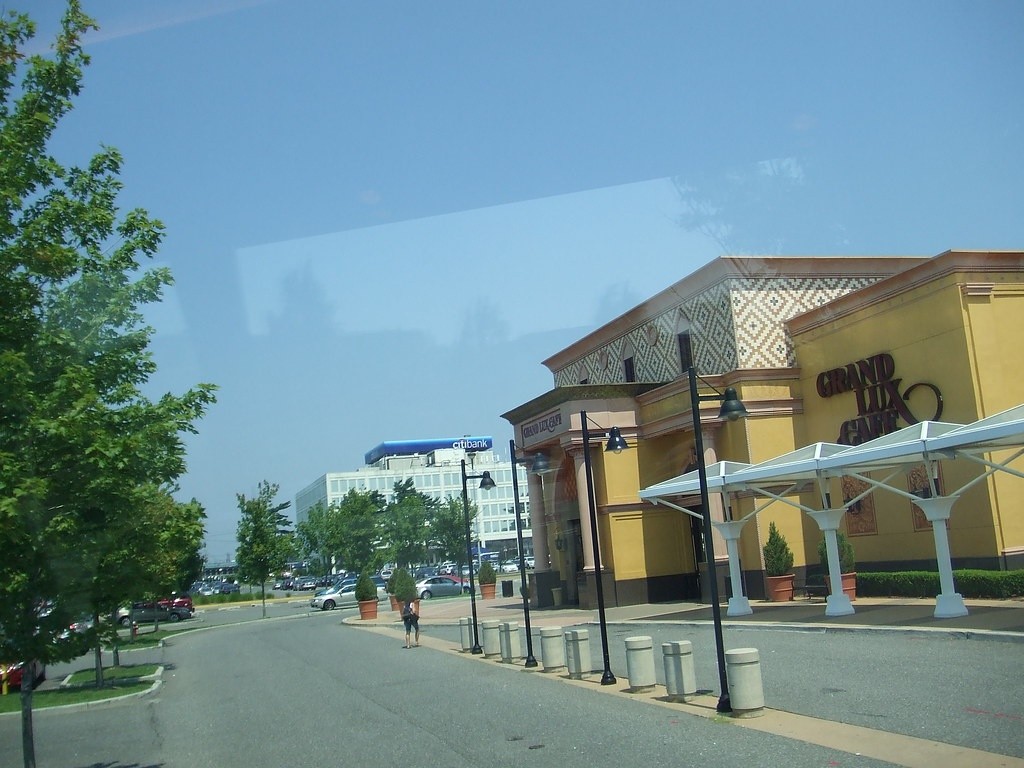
[310,583,388,611]
[413,576,476,602]
[0,647,45,689]
[192,546,535,597]
[32,586,192,645]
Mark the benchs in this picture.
[789,566,829,600]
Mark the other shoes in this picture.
[416,644,421,647]
[406,645,413,649]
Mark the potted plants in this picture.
[818,530,858,599]
[396,571,420,619]
[478,562,496,599]
[387,570,405,610]
[355,570,379,620]
[762,522,799,602]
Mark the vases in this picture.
[552,587,563,605]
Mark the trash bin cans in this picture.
[501,580,513,597]
[551,588,562,606]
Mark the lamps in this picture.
[555,536,562,552]
[910,487,929,505]
[844,495,861,515]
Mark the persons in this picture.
[402,594,421,649]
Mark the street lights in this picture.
[688,363,751,714]
[509,438,552,668]
[460,459,497,654]
[579,409,630,686]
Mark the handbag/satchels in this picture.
[410,612,420,623]
